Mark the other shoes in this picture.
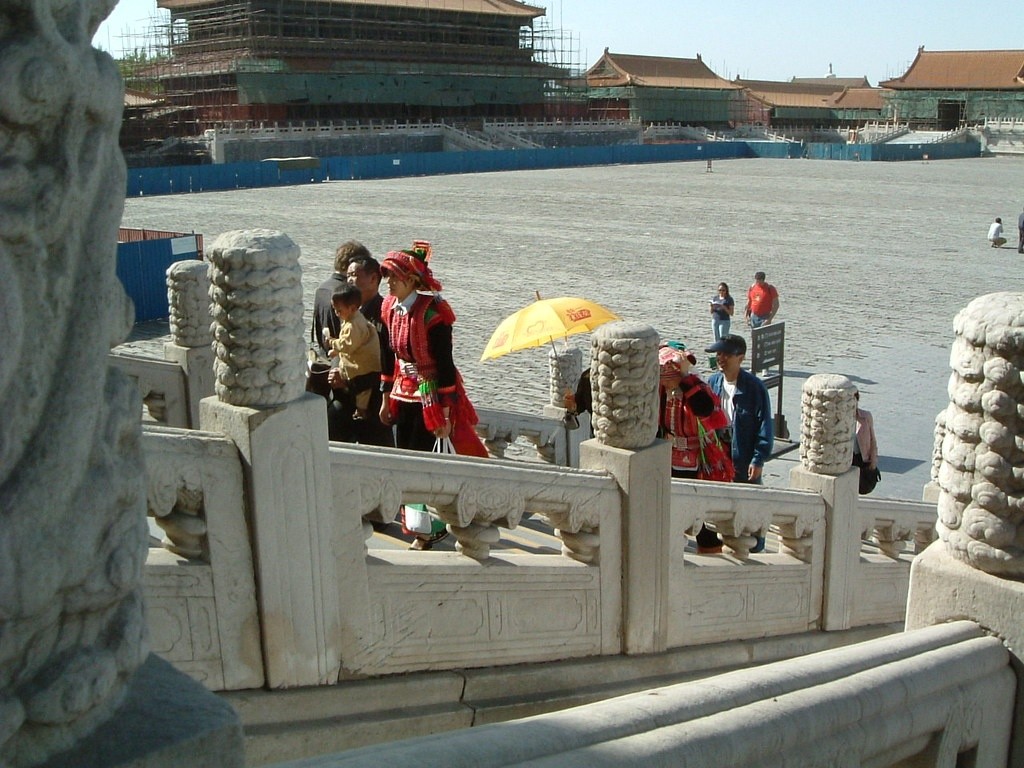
[432,527,449,543]
[698,546,722,555]
[762,367,769,376]
[409,536,434,551]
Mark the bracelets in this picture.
[444,417,449,421]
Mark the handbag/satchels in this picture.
[406,436,456,534]
[696,419,735,483]
[305,349,334,397]
[859,463,882,495]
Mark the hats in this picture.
[705,335,747,354]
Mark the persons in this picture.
[987,217,1007,248]
[658,341,734,554]
[853,390,876,495]
[562,367,595,438]
[1017,206,1024,253]
[703,334,774,553]
[379,239,489,550]
[710,282,735,341]
[744,272,779,378]
[313,239,395,448]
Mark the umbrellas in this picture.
[480,291,619,389]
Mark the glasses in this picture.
[718,289,726,292]
